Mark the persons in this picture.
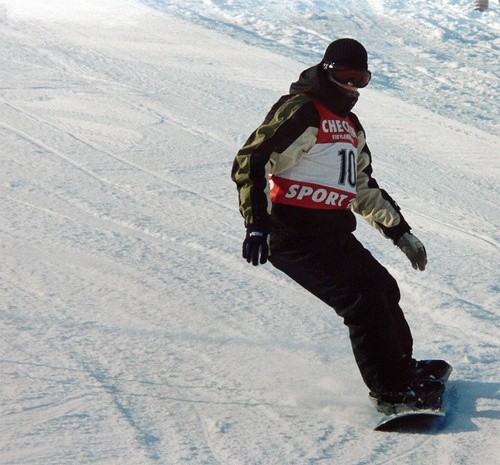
[230,39,446,415]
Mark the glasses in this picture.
[322,62,372,87]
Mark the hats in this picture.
[323,37,368,69]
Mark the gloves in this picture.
[243,219,272,266]
[396,230,428,271]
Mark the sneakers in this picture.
[406,359,450,380]
[376,377,445,415]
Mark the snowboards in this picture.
[373,359,453,430]
